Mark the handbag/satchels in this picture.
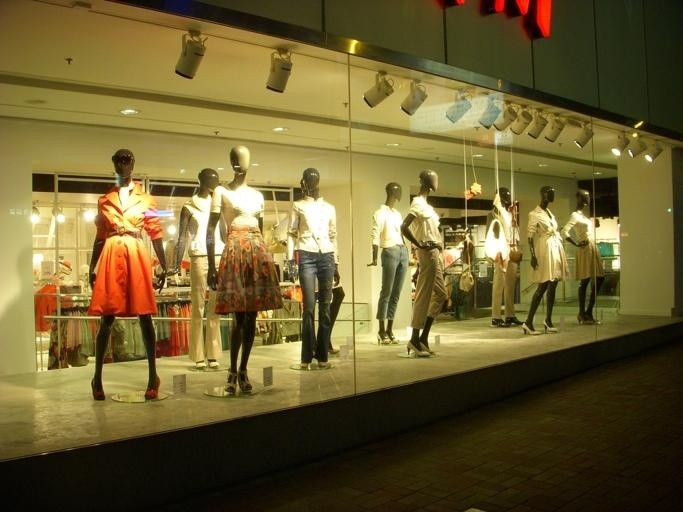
[458,272,474,292]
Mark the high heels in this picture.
[389,335,398,344]
[378,333,392,344]
[543,319,558,334]
[408,341,432,356]
[577,314,596,325]
[522,323,543,335]
[420,342,434,353]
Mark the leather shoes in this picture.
[300,363,310,368]
[197,362,207,368]
[224,368,238,393]
[91,377,105,400]
[318,361,330,368]
[506,317,524,325]
[328,348,340,354]
[238,370,252,391]
[493,319,510,327]
[144,376,160,400]
[208,360,220,367]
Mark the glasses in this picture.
[112,154,134,165]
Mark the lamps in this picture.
[445,91,472,123]
[478,96,594,149]
[30,204,41,218]
[174,28,208,81]
[362,69,396,109]
[610,130,664,162]
[265,49,293,94]
[51,206,65,222]
[400,79,429,117]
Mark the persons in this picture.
[206,144,284,392]
[299,180,345,357]
[400,169,448,356]
[367,182,409,344]
[561,190,604,326]
[287,168,340,369]
[165,168,228,368]
[88,149,166,399]
[485,187,526,328]
[522,186,569,336]
